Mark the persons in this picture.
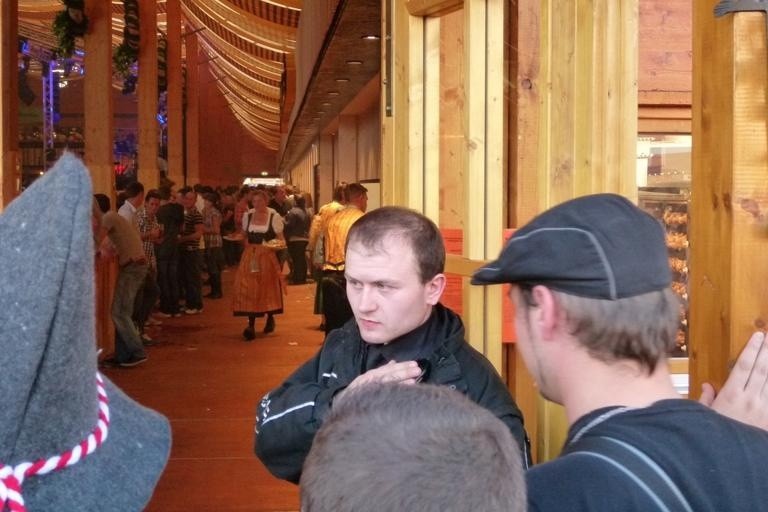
[470,193,768,512]
[254,205,533,485]
[136,184,369,340]
[94,194,148,367]
[298,382,528,512]
[117,181,161,346]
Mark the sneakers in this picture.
[119,291,224,367]
[243,326,256,340]
[264,320,275,333]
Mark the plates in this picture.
[222,236,244,241]
[263,246,289,250]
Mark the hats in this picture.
[1,153,174,511]
[470,194,672,300]
